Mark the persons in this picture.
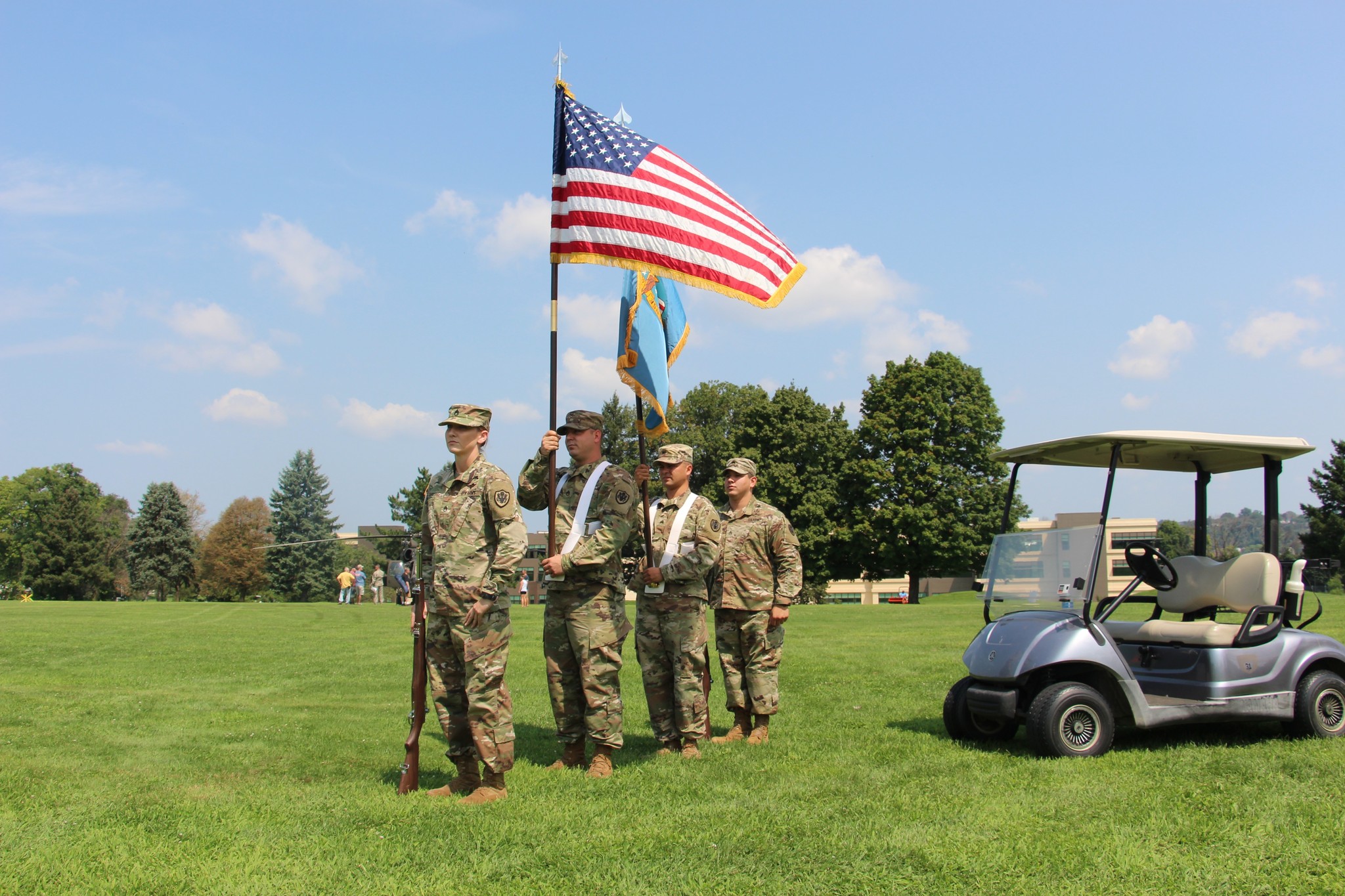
[401,568,411,606]
[628,444,724,758]
[355,564,368,604]
[370,564,384,606]
[516,410,641,780]
[705,457,802,746]
[521,570,529,605]
[418,404,529,806]
[349,568,356,604]
[519,577,527,607]
[336,568,355,605]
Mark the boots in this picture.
[656,738,681,756]
[426,760,482,798]
[746,714,769,744]
[681,738,701,760]
[545,739,587,772]
[586,743,613,778]
[457,766,507,804]
[711,712,753,743]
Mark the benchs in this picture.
[1092,552,1286,648]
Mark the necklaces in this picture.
[455,470,463,475]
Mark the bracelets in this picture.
[480,591,498,602]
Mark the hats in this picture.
[652,444,693,467]
[556,410,604,436]
[438,404,492,432]
[357,564,364,568]
[722,458,756,477]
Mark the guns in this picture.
[699,644,714,743]
[398,542,431,795]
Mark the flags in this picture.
[606,104,691,442]
[543,46,808,308]
